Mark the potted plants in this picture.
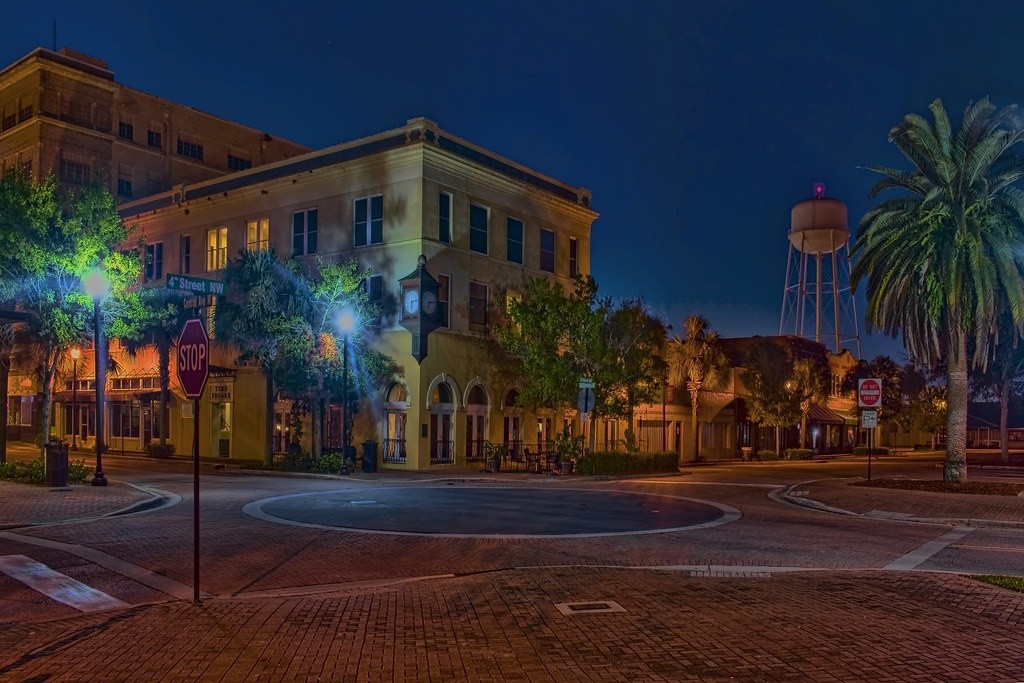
[487,444,506,472]
[547,425,586,474]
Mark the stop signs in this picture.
[176,318,210,400]
[859,379,881,407]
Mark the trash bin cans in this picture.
[361,440,379,473]
[43,444,70,486]
[742,447,753,462]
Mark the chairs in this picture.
[524,448,541,470]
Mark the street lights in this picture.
[82,270,110,486]
[70,346,80,451]
[335,309,353,476]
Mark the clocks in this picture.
[422,291,436,314]
[404,291,418,313]
